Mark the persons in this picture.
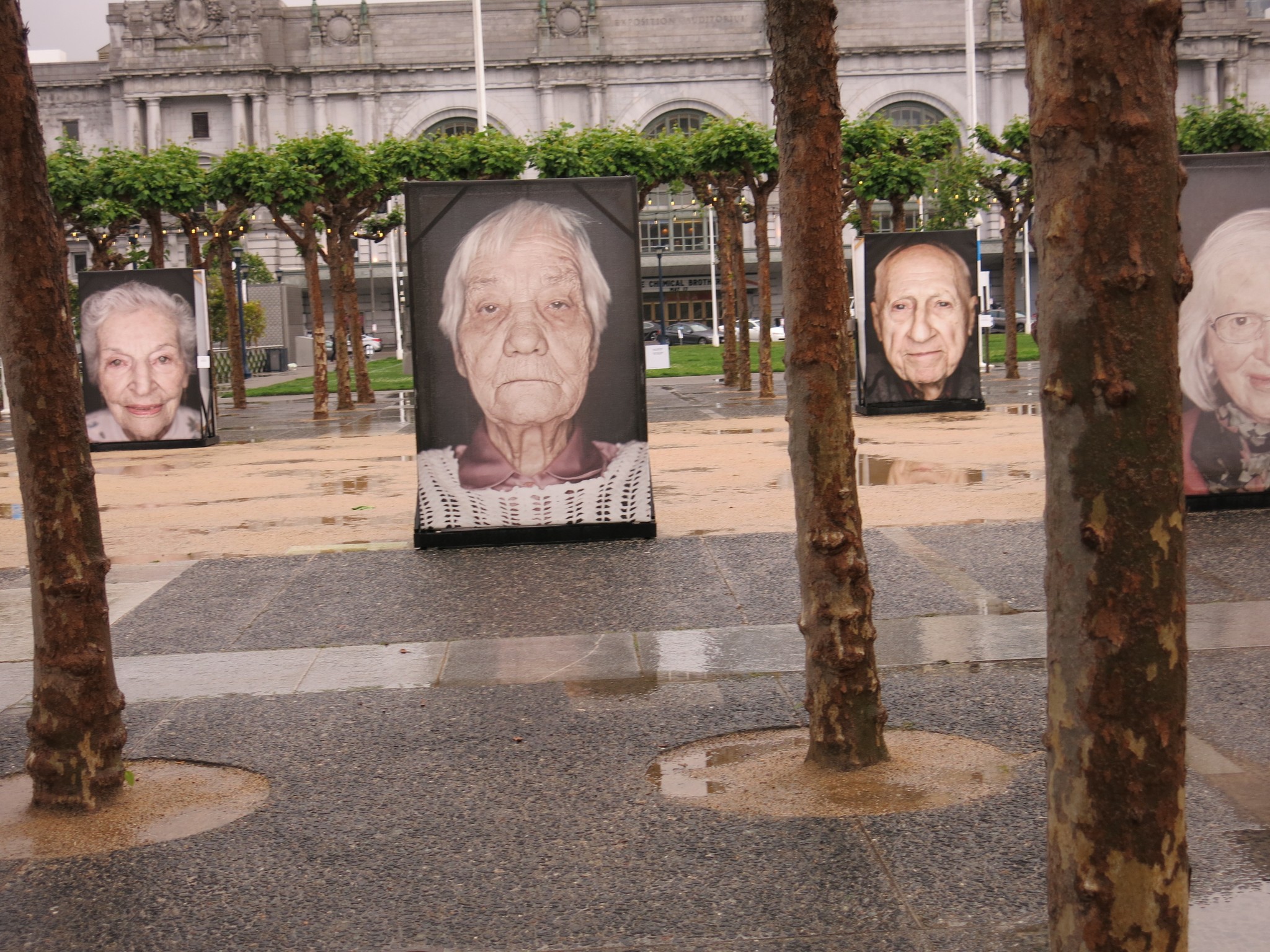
[1178,206,1270,495]
[79,281,210,444]
[417,199,655,530]
[867,242,982,404]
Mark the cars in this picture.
[305,330,382,361]
[657,322,725,346]
[718,318,786,342]
[643,320,661,341]
[982,308,1026,334]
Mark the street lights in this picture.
[231,246,252,379]
[128,225,140,270]
[653,245,666,344]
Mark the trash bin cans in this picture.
[265,347,288,372]
